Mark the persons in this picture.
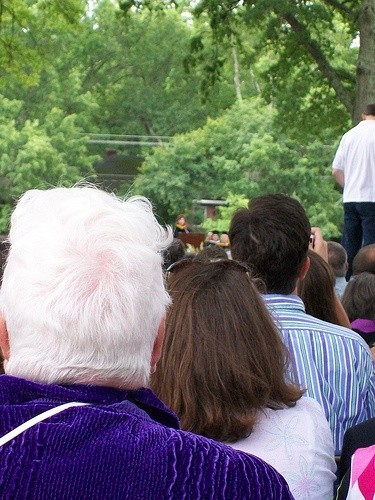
[1,186,297,500]
[330,104,375,282]
[298,227,352,330]
[326,240,348,304]
[228,192,375,463]
[335,416,375,499]
[350,243,375,278]
[147,257,338,500]
[159,213,232,261]
[340,272,375,372]
